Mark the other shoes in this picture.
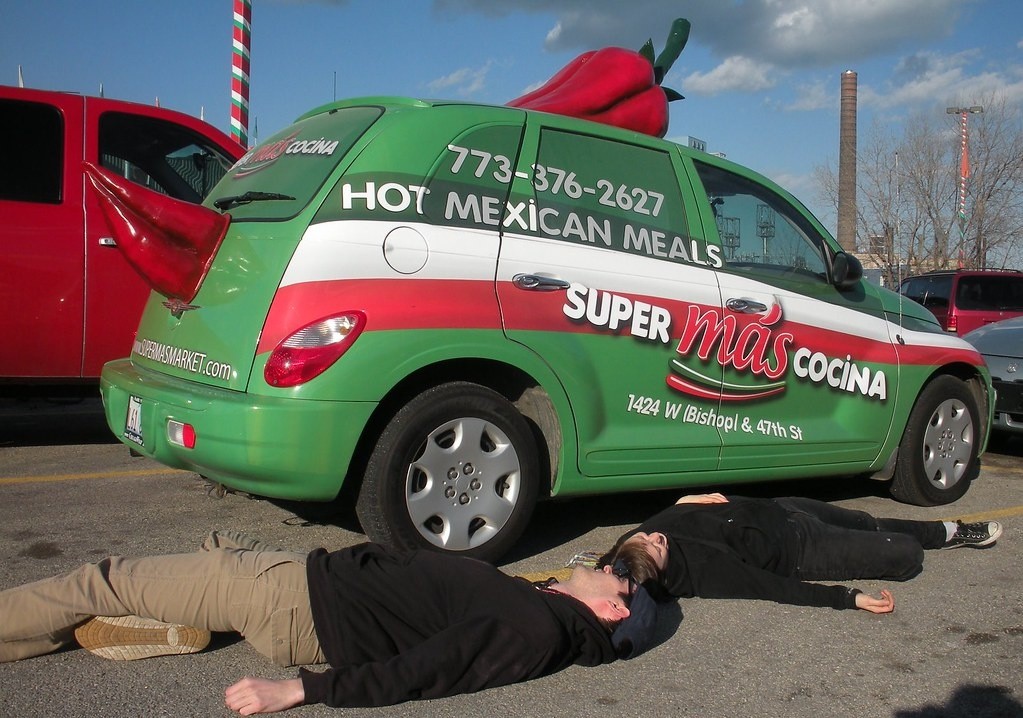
[75,614,210,660]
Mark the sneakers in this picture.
[941,519,1004,551]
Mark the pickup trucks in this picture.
[0,85,249,401]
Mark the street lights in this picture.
[945,106,982,269]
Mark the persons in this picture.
[593,492,1003,614]
[0,526,661,715]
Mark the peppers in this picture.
[490,17,691,138]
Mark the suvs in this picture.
[894,266,1023,338]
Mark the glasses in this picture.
[612,568,634,608]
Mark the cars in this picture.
[959,315,1023,434]
[97,95,997,571]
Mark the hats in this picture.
[608,575,681,660]
